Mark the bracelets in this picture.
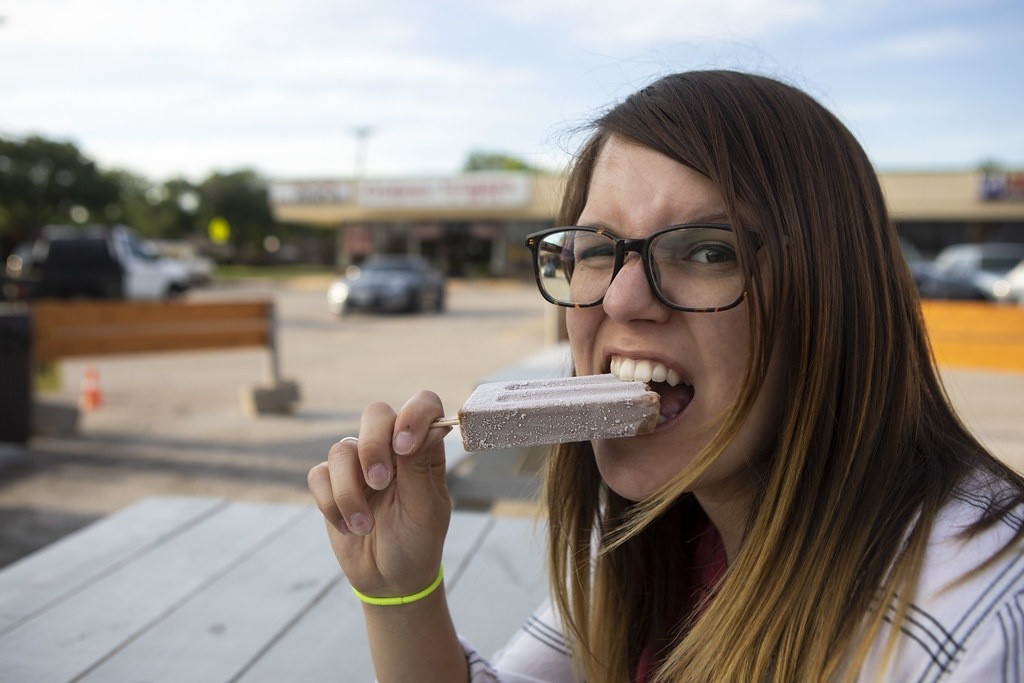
[350,562,443,605]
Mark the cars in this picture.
[18,225,188,302]
[150,241,214,293]
[332,255,445,312]
[899,242,1024,303]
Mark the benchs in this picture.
[23,300,298,426]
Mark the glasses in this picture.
[525,223,769,312]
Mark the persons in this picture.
[306,70,1024,683]
